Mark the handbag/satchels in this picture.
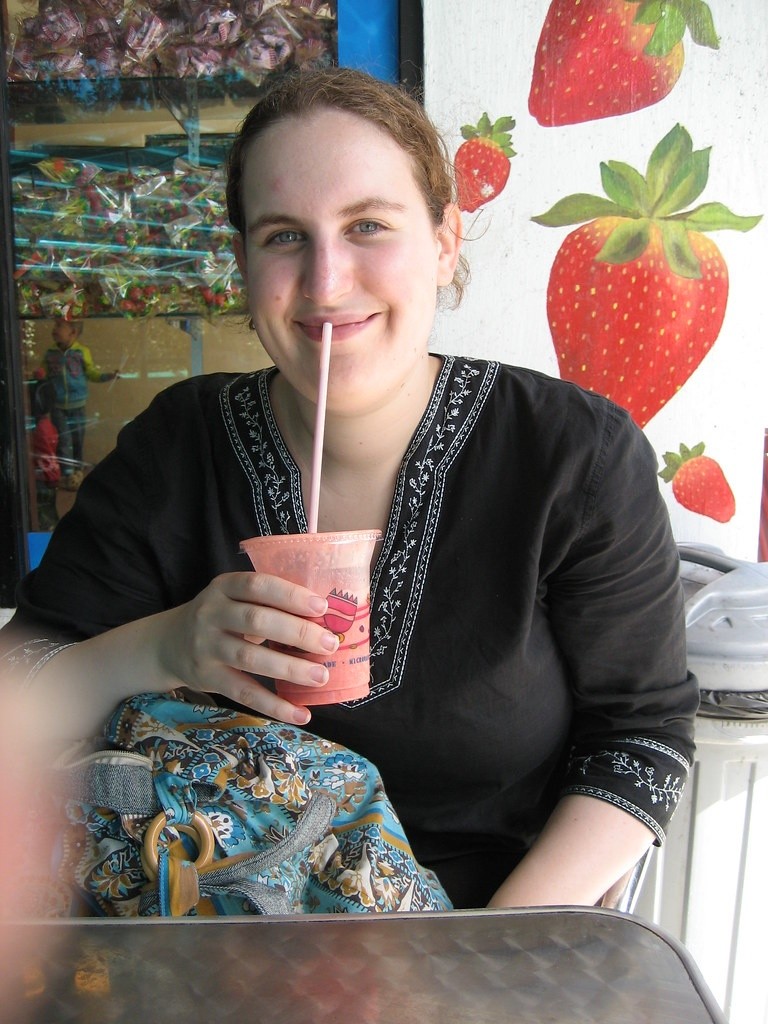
[43,687,452,919]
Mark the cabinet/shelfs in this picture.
[0,0,424,624]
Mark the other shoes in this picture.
[65,473,79,490]
[75,470,84,483]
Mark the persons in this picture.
[29,316,121,529]
[0,67,701,921]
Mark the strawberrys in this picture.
[17,159,239,312]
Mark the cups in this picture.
[237,527,384,707]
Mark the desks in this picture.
[0,904,728,1024]
